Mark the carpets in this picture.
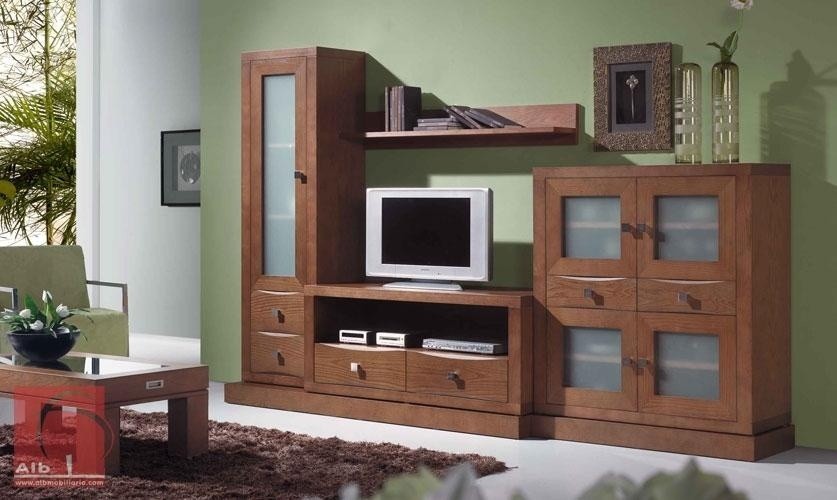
[0,408,519,500]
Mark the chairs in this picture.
[0,244,138,355]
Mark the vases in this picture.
[676,60,740,163]
[6,329,75,361]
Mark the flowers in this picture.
[707,0,756,62]
[0,292,95,343]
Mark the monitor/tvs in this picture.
[363,185,495,292]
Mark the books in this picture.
[384,85,524,132]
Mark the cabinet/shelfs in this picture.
[300,283,533,441]
[533,163,796,463]
[224,45,366,409]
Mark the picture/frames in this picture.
[591,39,675,154]
[157,128,200,208]
